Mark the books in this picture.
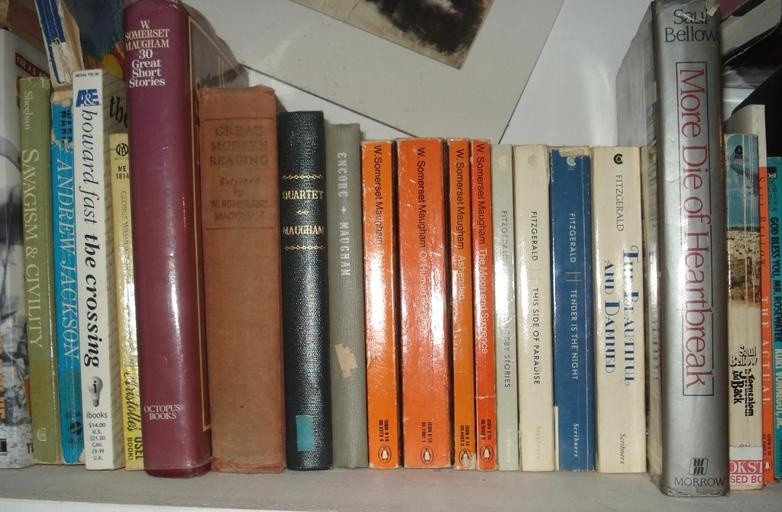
[0,2,781,498]
